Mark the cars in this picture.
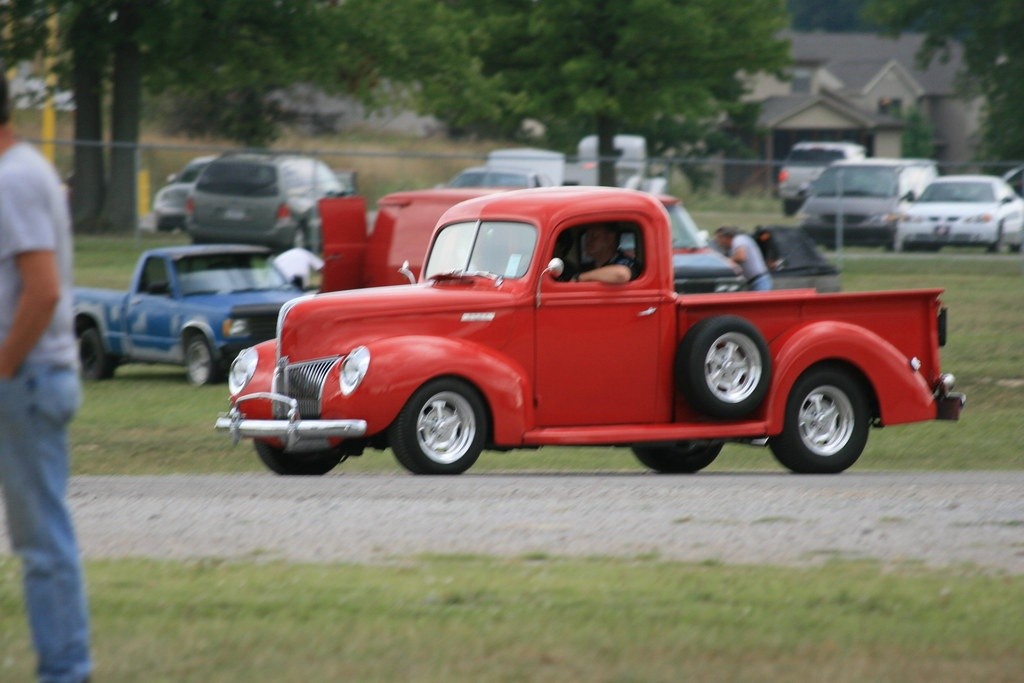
[1002,168,1024,200]
[442,165,550,194]
[622,194,747,296]
[890,173,1024,255]
[150,152,218,232]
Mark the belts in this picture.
[747,271,768,285]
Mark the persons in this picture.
[0,71,90,683]
[551,230,583,282]
[274,246,326,289]
[713,224,788,292]
[570,221,635,284]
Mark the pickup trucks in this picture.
[214,182,963,475]
[70,243,305,384]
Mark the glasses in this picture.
[587,232,605,240]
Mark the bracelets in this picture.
[575,271,580,282]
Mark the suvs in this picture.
[798,158,942,245]
[181,150,351,253]
[778,142,868,215]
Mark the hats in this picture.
[754,225,768,238]
